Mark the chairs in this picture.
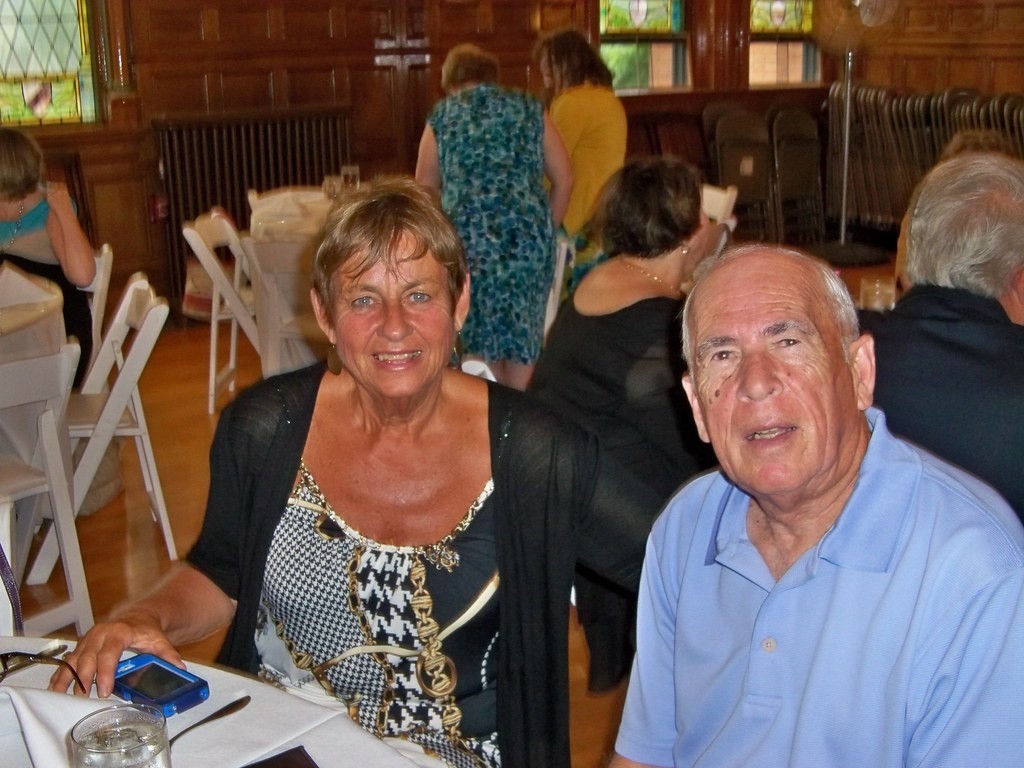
[701,102,826,251]
[0,165,258,671]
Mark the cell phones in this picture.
[240,745,319,768]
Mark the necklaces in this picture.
[614,257,683,299]
[0,199,25,250]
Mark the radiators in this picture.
[151,102,352,303]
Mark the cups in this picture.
[861,275,895,310]
[71,704,172,768]
[322,166,359,198]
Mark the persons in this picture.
[48,176,667,768]
[418,30,627,392]
[0,127,96,393]
[853,152,1024,527]
[526,158,722,689]
[894,130,1013,297]
[610,245,1024,768]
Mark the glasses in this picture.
[0,644,88,693]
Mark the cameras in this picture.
[111,653,210,719]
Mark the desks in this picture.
[1,637,453,768]
